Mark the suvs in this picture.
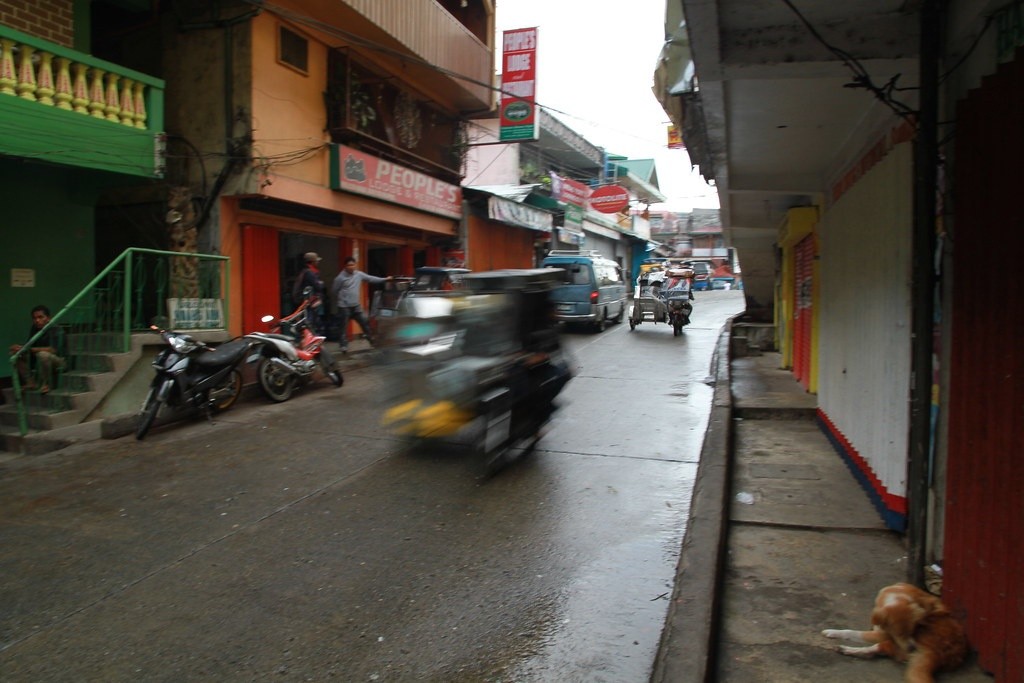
[540,248,630,333]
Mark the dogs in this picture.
[820,582,967,683]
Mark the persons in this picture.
[289,251,327,333]
[330,256,394,354]
[6,306,66,397]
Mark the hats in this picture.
[302,253,322,261]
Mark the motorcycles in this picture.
[679,258,713,291]
[136,326,253,440]
[243,299,345,403]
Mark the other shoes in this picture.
[341,346,347,352]
[21,383,38,392]
[39,384,50,394]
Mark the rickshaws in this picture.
[629,256,693,336]
[384,267,585,464]
